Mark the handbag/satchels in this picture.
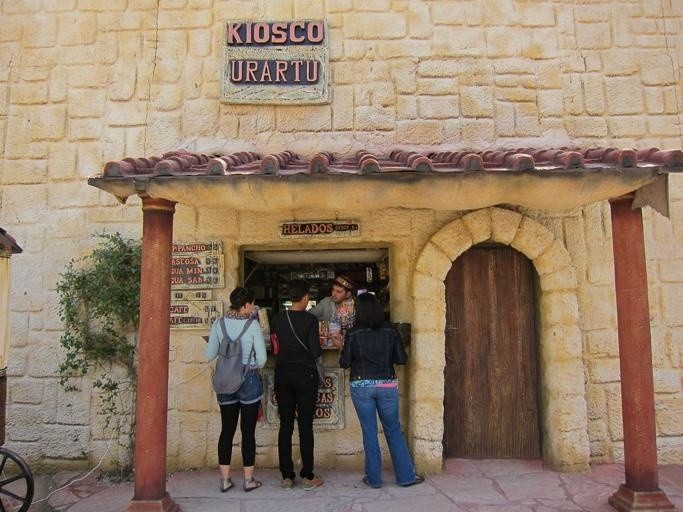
[314,358,324,386]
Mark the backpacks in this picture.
[213,318,255,395]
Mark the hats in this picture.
[335,274,355,290]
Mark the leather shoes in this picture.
[402,475,425,487]
[363,475,381,488]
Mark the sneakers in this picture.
[220,479,233,491]
[243,477,262,491]
[303,477,322,491]
[281,478,294,490]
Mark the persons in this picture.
[338,291,426,489]
[202,284,268,493]
[266,278,325,491]
[306,273,357,327]
